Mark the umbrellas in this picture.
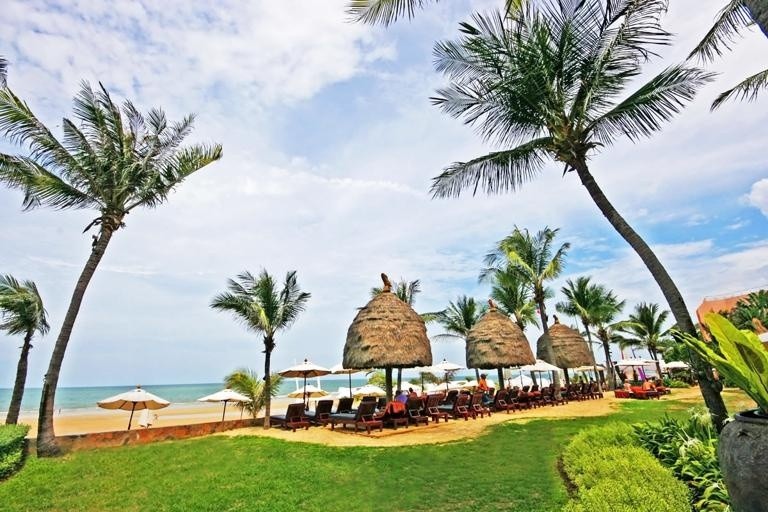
[277,359,363,410]
[664,361,689,372]
[521,357,561,388]
[416,359,465,392]
[96,389,171,430]
[199,387,251,422]
[613,357,659,376]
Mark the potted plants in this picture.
[668,310,767,512]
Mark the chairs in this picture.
[270,402,308,431]
[304,398,333,427]
[330,384,603,433]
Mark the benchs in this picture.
[614,386,665,399]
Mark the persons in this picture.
[479,373,495,405]
[633,369,639,381]
[508,377,512,388]
[578,376,583,383]
[642,377,656,392]
[623,379,631,392]
[408,387,417,399]
[394,390,408,404]
